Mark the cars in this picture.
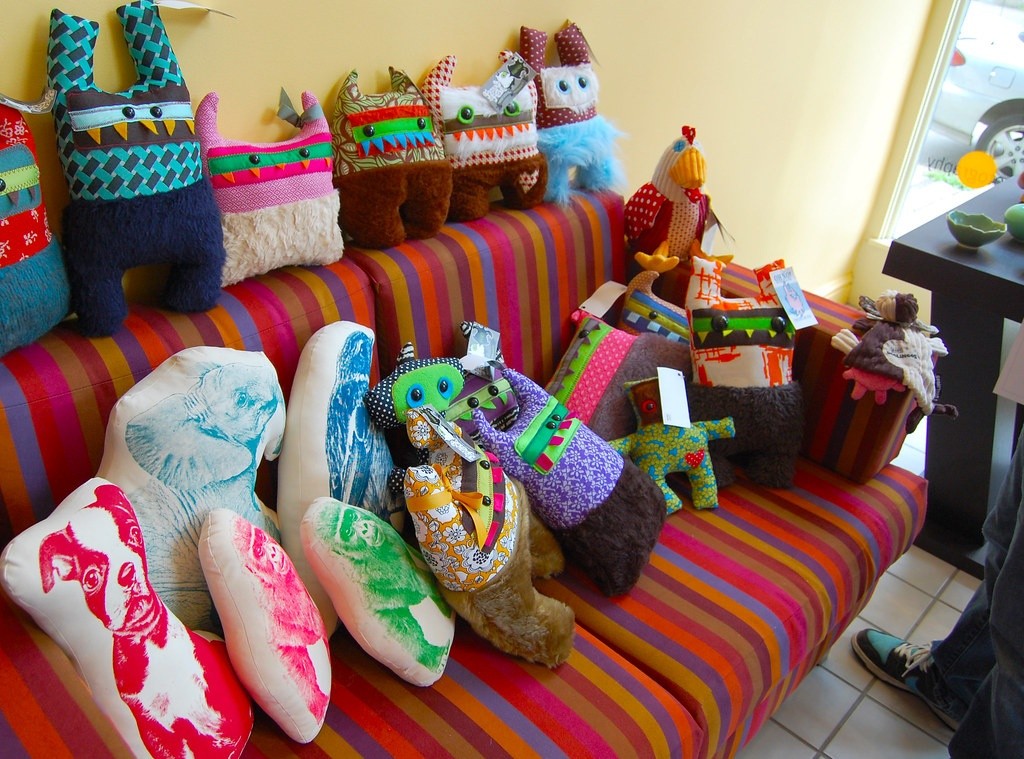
[931,0,1024,185]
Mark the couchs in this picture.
[0,190,929,759]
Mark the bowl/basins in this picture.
[946,209,1008,249]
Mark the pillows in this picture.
[276,320,404,640]
[198,508,332,744]
[0,476,256,759]
[94,346,286,634]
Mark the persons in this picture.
[851,424,1024,758]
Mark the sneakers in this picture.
[851,628,964,732]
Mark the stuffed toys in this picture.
[1,306,810,759]
[0,1,959,363]
[624,123,710,275]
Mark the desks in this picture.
[883,171,1024,553]
